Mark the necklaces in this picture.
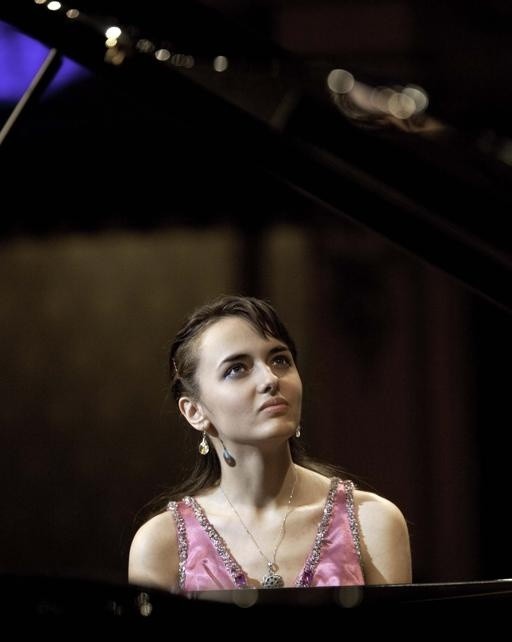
[215,465,299,589]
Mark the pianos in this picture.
[1,0,511,641]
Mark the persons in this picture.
[122,292,418,591]
